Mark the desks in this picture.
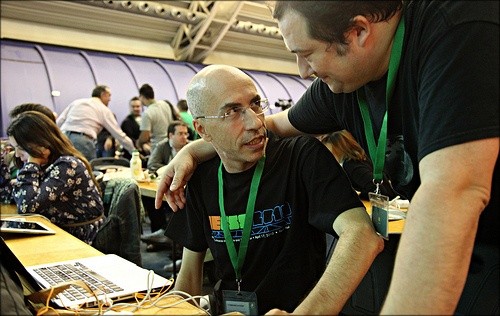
[0,203,208,316]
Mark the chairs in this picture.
[89,181,145,266]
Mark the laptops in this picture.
[0,235,173,310]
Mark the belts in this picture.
[71,131,95,141]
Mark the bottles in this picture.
[130,149,144,181]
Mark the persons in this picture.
[1,103,57,170]
[147,120,194,173]
[155,0,500,316]
[0,111,107,245]
[136,83,197,154]
[320,130,384,201]
[172,65,385,316]
[56,84,150,161]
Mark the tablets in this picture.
[0,219,56,235]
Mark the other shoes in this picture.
[164,259,181,272]
[146,243,160,252]
[141,230,172,245]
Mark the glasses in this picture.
[197,98,270,125]
